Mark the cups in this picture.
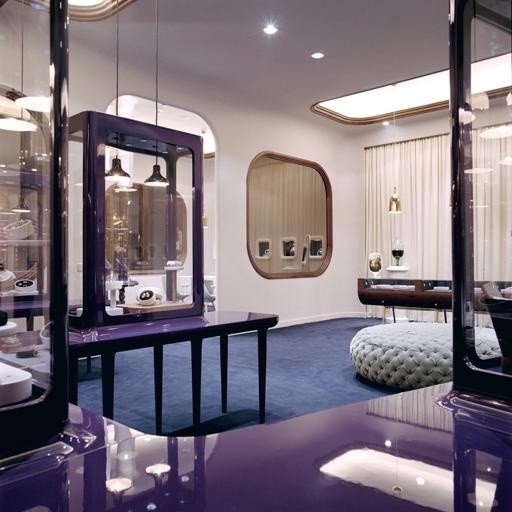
[391,238,405,266]
[368,253,382,280]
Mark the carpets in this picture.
[166,408,285,438]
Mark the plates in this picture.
[433,287,449,292]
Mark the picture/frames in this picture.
[255,238,272,260]
[279,236,297,259]
[308,235,324,259]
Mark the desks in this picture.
[2,383,511,512]
[65,309,279,435]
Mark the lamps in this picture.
[0,0,39,133]
[387,82,402,215]
[103,2,134,183]
[143,1,172,188]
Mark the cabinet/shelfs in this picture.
[356,277,512,323]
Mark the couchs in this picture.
[350,321,502,389]
